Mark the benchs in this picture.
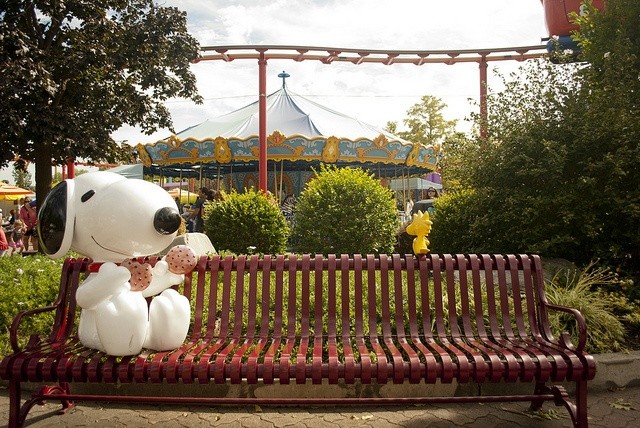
[1,254,596,426]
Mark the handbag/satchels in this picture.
[32,226,38,237]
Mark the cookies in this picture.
[165,244,199,274]
[120,257,153,292]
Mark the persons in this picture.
[186,187,226,233]
[0,208,16,252]
[10,221,26,255]
[425,187,439,199]
[19,196,38,251]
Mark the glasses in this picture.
[25,201,30,203]
[428,190,436,192]
